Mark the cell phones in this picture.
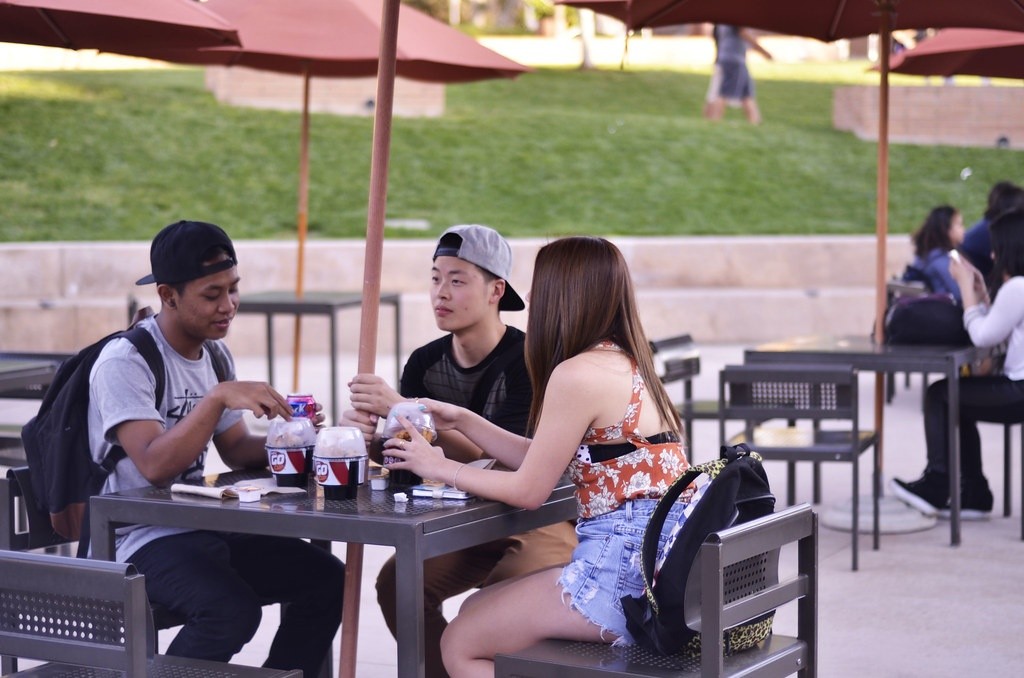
[947,249,960,263]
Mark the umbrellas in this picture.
[2,1,531,678]
[554,0,1024,498]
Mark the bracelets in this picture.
[452,462,468,492]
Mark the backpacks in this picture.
[903,253,945,292]
[21,327,228,540]
[621,443,776,658]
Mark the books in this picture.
[408,483,468,500]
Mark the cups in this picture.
[311,454,365,501]
[377,437,432,486]
[265,443,313,488]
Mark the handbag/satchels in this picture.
[870,293,972,346]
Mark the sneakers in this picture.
[940,491,992,519]
[893,477,947,518]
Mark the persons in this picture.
[901,182,1023,299]
[706,24,775,125]
[349,225,575,678]
[887,210,1022,519]
[383,238,699,677]
[88,219,347,678]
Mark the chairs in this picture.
[0,334,884,678]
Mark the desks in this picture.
[234,289,401,427]
[748,339,991,547]
[90,470,573,678]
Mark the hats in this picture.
[433,225,525,311]
[136,220,236,285]
